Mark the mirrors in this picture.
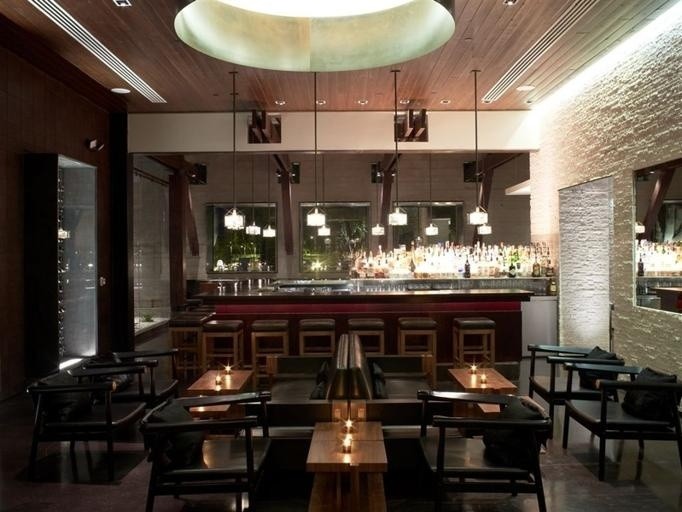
[132,152,530,276]
[633,162,681,314]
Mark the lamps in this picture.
[170,0,456,73]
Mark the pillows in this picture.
[621,367,676,421]
[485,398,542,464]
[37,369,91,425]
[153,399,205,467]
[576,346,616,391]
[370,361,388,399]
[84,352,133,395]
[310,360,328,400]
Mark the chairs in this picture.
[417,387,555,511]
[564,362,679,483]
[137,391,272,512]
[527,344,625,439]
[27,367,149,479]
[81,349,179,411]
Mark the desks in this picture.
[448,367,519,395]
[306,422,390,512]
[186,368,255,396]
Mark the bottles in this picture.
[546,277,557,296]
[348,237,555,277]
[635,237,682,277]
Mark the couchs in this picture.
[272,333,348,399]
[349,333,431,399]
